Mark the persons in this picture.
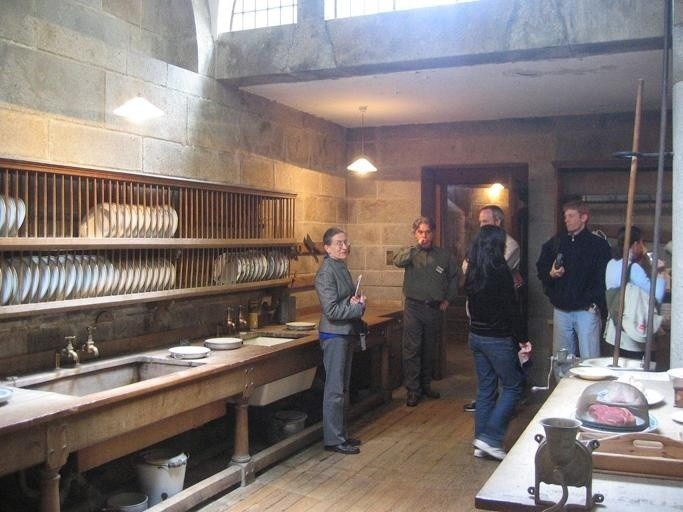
[461,205,528,412]
[536,198,610,384]
[310,226,368,456]
[392,216,461,408]
[601,225,664,361]
[463,224,532,461]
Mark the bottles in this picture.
[225,299,260,334]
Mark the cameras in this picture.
[555,252,568,270]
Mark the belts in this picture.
[405,297,440,306]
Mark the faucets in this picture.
[221,305,240,332]
[75,324,101,360]
[51,335,79,373]
[233,299,249,329]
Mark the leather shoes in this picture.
[324,437,360,455]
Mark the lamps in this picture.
[112,1,168,127]
[347,105,379,174]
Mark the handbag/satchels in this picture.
[606,284,626,330]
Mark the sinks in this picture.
[5,355,208,397]
[227,331,320,408]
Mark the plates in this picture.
[286,322,316,330]
[0,195,178,239]
[169,345,210,360]
[0,251,290,308]
[595,388,663,406]
[205,336,242,352]
[572,413,661,438]
[569,366,615,381]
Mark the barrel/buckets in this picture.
[271,410,307,439]
[104,491,148,511]
[130,446,190,504]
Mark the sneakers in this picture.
[472,437,506,459]
[422,390,440,399]
[463,401,477,412]
[406,394,418,407]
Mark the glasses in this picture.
[336,241,351,246]
